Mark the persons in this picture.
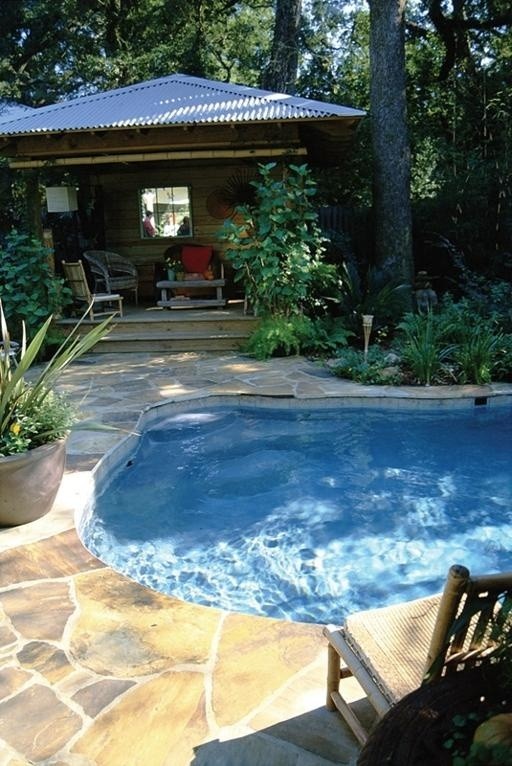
[178,216,189,236]
[143,210,156,237]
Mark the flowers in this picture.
[162,257,182,271]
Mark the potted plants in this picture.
[0,294,142,527]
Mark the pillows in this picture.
[180,246,214,273]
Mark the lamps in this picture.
[46,185,79,214]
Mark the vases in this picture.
[167,269,176,281]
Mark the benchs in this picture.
[156,279,227,311]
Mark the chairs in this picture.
[61,260,124,323]
[85,251,138,308]
[317,564,512,750]
[153,243,226,299]
